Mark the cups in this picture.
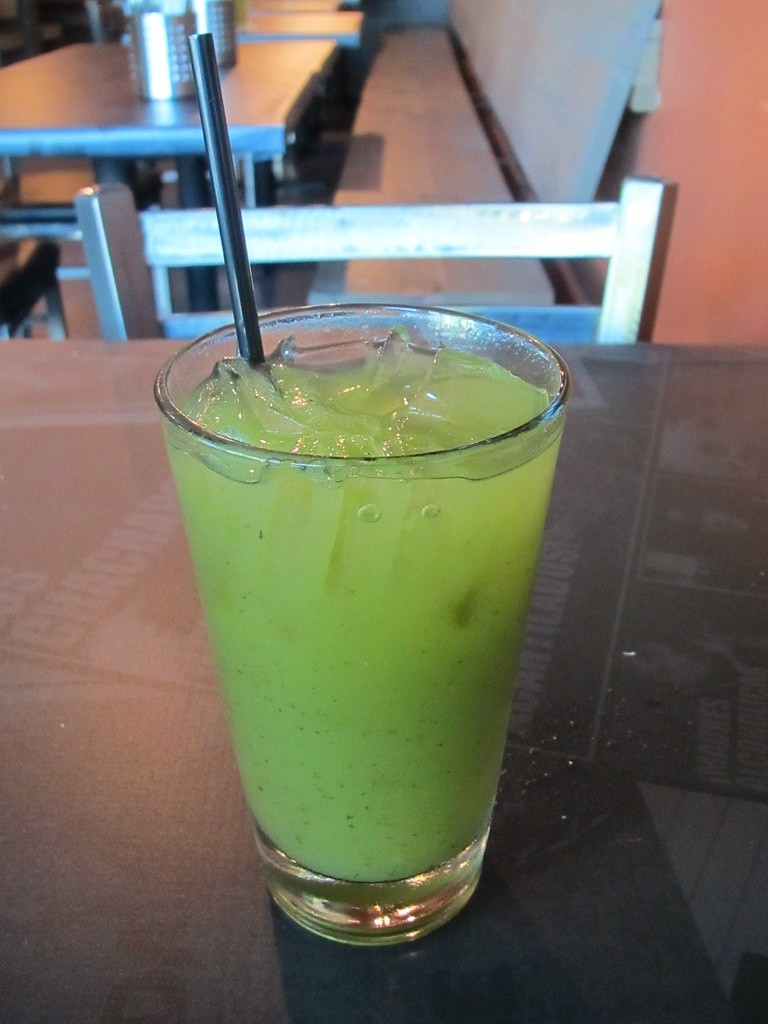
[153,304,568,946]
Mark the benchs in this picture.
[308,1,660,306]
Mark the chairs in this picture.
[0,176,680,342]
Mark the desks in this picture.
[0,1,366,312]
[0,341,768,1024]
